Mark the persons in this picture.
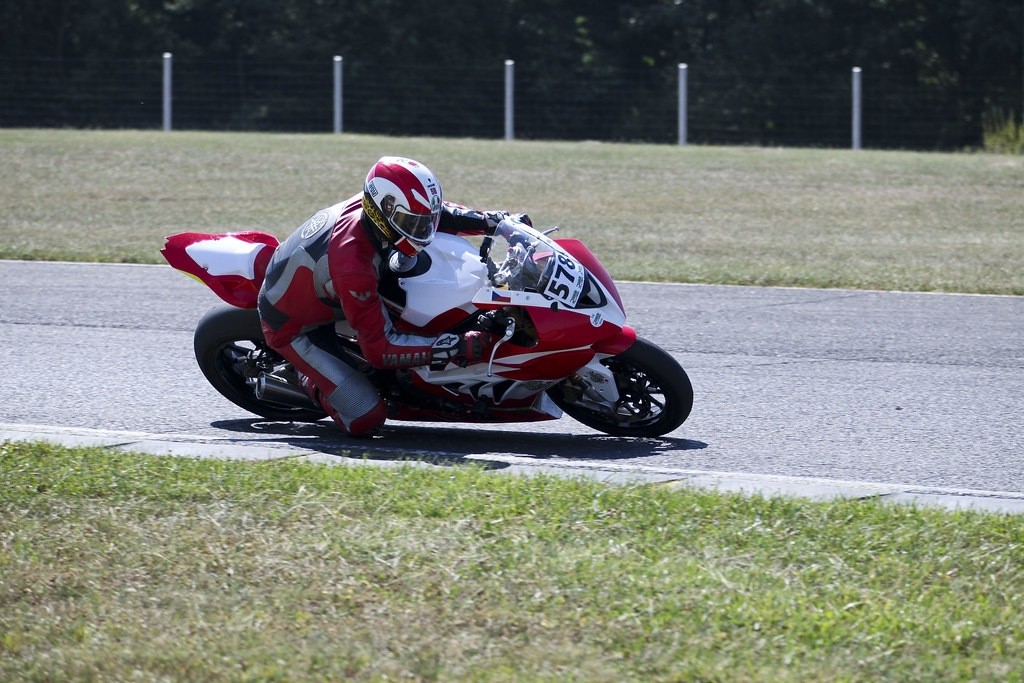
[256,155,534,436]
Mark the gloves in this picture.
[458,331,492,361]
[506,213,533,227]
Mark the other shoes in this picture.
[299,376,320,407]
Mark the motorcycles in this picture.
[157,214,695,439]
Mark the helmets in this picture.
[364,156,443,257]
